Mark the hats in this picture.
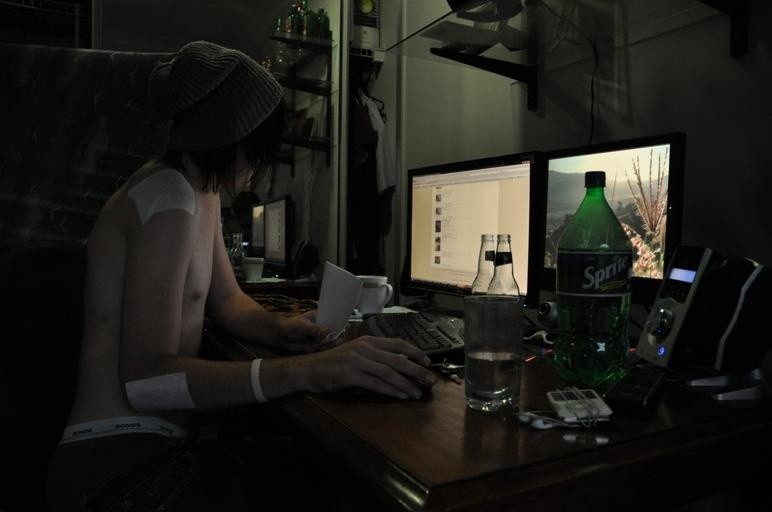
[146,38,285,151]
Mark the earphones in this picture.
[520,412,531,422]
[531,419,546,430]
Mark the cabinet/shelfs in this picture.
[248,32,333,166]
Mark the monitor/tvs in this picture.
[407,152,541,310]
[540,133,685,306]
[250,195,295,280]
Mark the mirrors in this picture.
[90,1,340,291]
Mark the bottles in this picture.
[229,232,246,284]
[556,172,634,387]
[275,0,331,39]
[472,234,520,299]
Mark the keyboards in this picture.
[365,312,465,365]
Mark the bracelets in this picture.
[246,355,271,405]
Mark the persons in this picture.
[223,190,266,259]
[44,41,440,511]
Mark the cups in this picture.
[242,257,264,282]
[463,294,523,414]
[354,275,394,316]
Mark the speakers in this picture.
[292,240,317,276]
[399,255,434,306]
[686,257,767,399]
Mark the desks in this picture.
[201,327,771,512]
